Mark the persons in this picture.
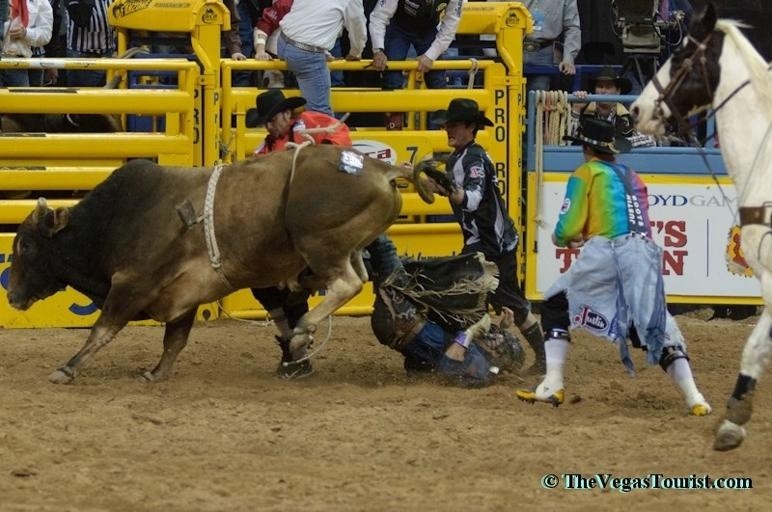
[398,98,548,377]
[243,86,355,381]
[285,231,529,390]
[0,1,582,131]
[564,65,657,150]
[509,113,714,418]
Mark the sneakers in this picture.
[688,402,712,416]
[514,386,567,408]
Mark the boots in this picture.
[273,334,314,383]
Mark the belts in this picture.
[279,29,327,54]
[523,38,558,54]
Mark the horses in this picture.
[625,0,772,452]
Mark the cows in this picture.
[4,142,457,386]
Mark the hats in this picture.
[244,86,307,129]
[561,114,633,155]
[581,66,633,95]
[428,97,495,127]
